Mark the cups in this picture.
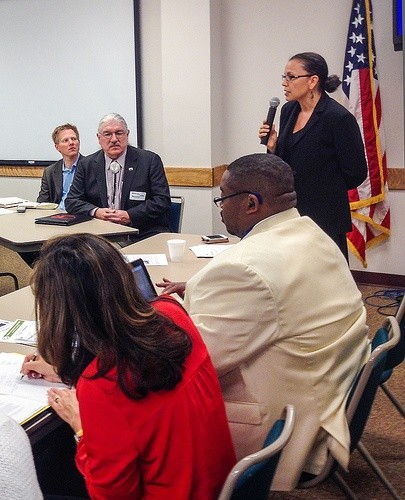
[167,239,186,263]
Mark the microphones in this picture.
[261,97,280,145]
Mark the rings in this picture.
[54,396,61,402]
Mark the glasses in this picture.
[281,72,322,83]
[213,191,263,208]
[99,129,128,138]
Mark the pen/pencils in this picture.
[20,350,38,379]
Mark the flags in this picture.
[340,0,391,270]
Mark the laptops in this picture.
[128,258,158,301]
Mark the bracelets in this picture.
[74,429,83,442]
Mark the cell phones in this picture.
[201,234,228,241]
[17,206,26,213]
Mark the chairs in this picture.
[220,288,405,500]
[120,196,185,234]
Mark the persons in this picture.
[156,152,372,491]
[64,113,171,248]
[20,233,236,500]
[258,53,369,266]
[36,124,87,209]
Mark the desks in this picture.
[0,198,138,251]
[0,233,236,461]
